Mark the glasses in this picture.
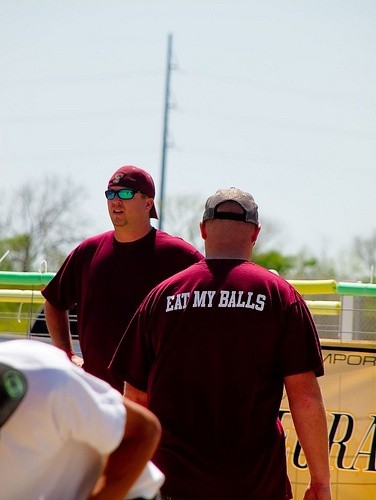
[105,188,141,200]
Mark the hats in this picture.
[202,187,259,226]
[108,165,158,219]
